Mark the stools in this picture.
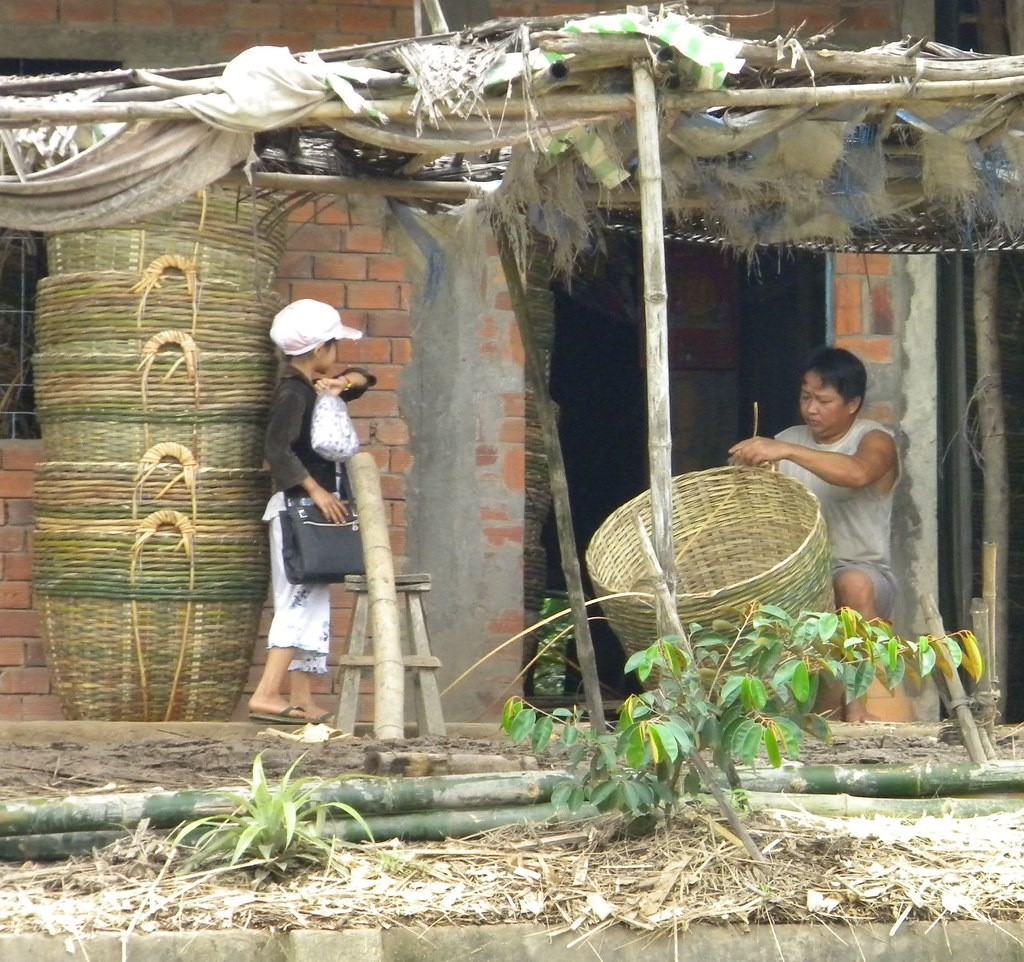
[335,574,447,738]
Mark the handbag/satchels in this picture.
[279,502,366,585]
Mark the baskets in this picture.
[32,188,284,721]
[585,464,838,717]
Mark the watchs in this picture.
[343,375,353,391]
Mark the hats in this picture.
[270,299,362,354]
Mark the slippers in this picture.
[248,705,319,724]
[317,711,335,723]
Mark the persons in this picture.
[728,347,902,720]
[247,298,377,725]
